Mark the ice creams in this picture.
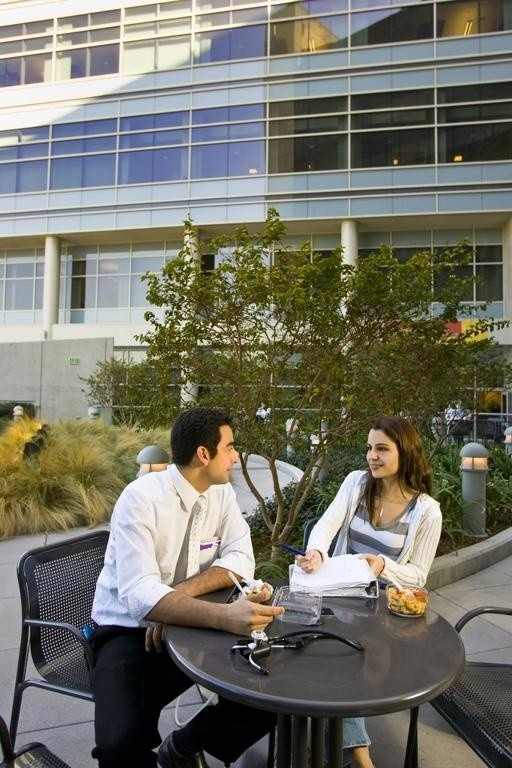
[239,576,274,606]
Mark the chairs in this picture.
[9,530,126,753]
[443,603,511,768]
[0,708,83,768]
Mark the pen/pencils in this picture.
[281,544,306,556]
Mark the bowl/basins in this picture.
[386,584,428,618]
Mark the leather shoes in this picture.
[155,729,211,768]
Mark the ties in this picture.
[186,496,206,581]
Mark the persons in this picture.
[83,407,285,768]
[297,416,444,767]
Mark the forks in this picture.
[388,576,419,599]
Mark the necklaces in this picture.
[377,485,410,517]
